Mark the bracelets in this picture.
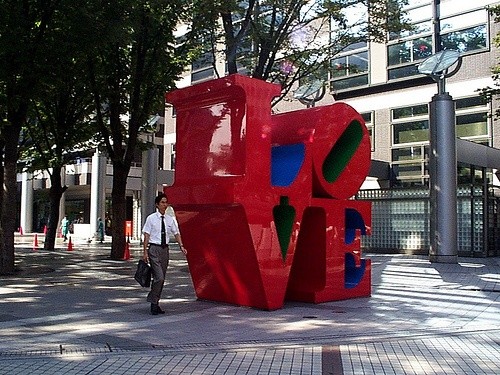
[180,246,184,249]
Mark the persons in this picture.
[142,194,187,315]
[61,215,71,242]
[96,217,104,243]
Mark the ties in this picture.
[160,216,167,249]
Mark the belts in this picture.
[151,244,168,247]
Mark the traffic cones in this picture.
[33,233,38,247]
[123,243,130,261]
[67,236,73,251]
[19,226,24,236]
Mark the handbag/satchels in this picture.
[134,259,151,287]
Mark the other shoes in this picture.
[151,305,165,314]
[147,297,157,304]
